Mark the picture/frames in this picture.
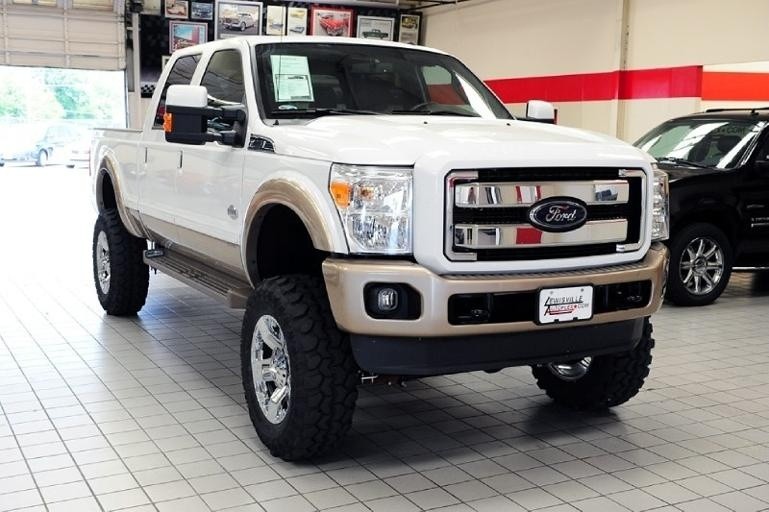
[162,0,422,70]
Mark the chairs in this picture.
[348,72,410,113]
[711,137,741,158]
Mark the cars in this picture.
[160,1,422,53]
[0,117,120,168]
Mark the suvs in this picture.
[631,102,769,304]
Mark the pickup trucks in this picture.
[90,35,681,464]
